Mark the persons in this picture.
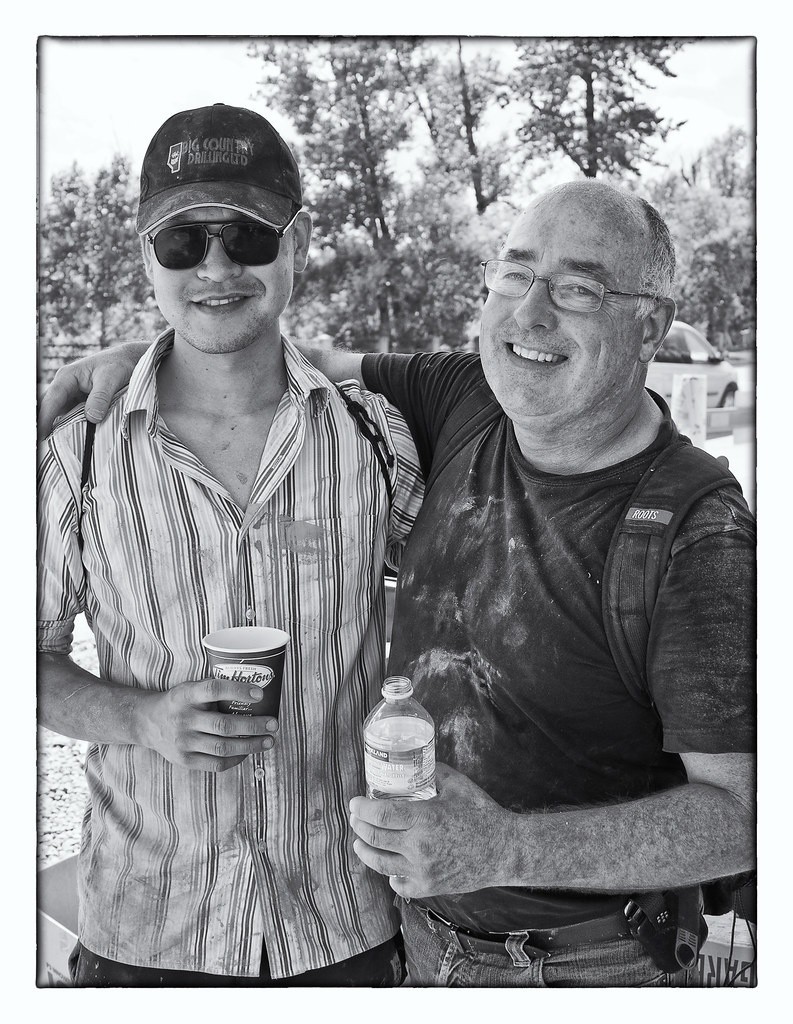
[37,104,756,987]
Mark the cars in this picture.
[644,319,739,413]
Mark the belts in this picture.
[420,908,635,947]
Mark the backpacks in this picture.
[421,385,756,916]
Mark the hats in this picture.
[137,104,303,236]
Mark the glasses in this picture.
[146,209,301,270]
[480,260,661,313]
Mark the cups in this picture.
[201,627,290,739]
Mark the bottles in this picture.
[364,675,437,802]
